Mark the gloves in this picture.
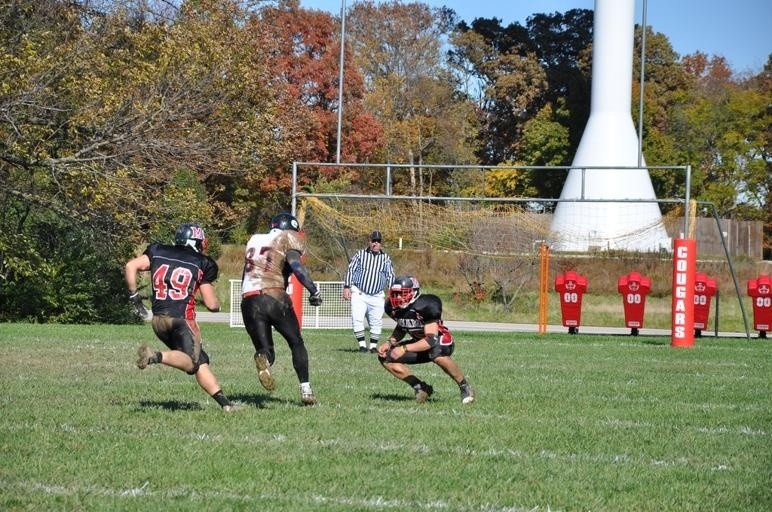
[129,290,150,315]
[308,292,322,306]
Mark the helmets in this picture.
[270,213,299,234]
[176,223,207,255]
[388,274,421,310]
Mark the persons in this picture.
[125,223,243,412]
[241,213,323,405]
[378,274,474,405]
[343,231,396,354]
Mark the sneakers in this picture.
[220,401,251,415]
[370,346,378,354]
[255,352,275,391]
[460,382,474,406]
[417,380,432,404]
[358,345,367,352]
[136,342,154,369]
[298,382,318,406]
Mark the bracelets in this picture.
[401,345,406,353]
[386,340,392,346]
[403,344,407,352]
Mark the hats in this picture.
[371,231,381,241]
